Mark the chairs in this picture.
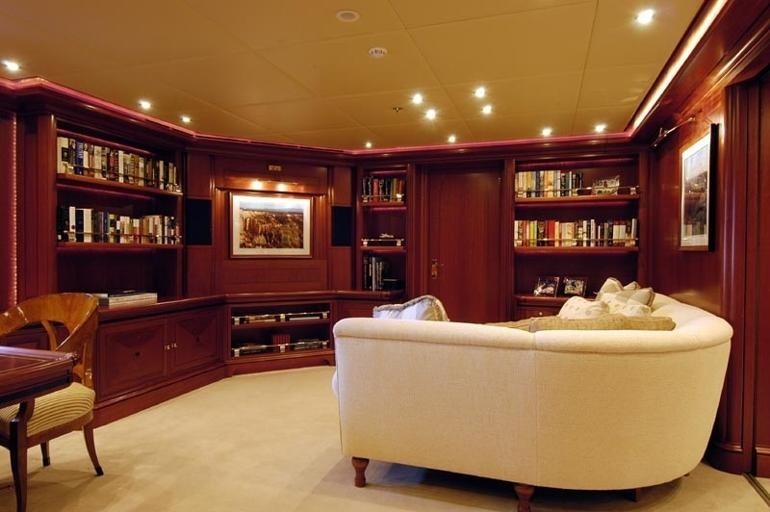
[0,292,104,512]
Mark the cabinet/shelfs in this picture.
[0,0,770,474]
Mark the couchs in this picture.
[333,288,735,512]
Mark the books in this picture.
[512,169,638,249]
[271,332,292,354]
[93,290,158,304]
[360,173,407,204]
[362,256,384,291]
[53,135,181,248]
